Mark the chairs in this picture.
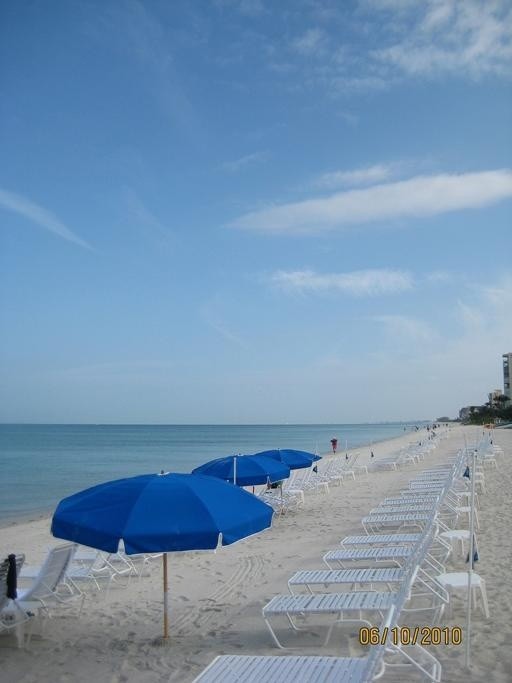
[192,424,503,683]
[2,540,164,648]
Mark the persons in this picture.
[330,437,338,455]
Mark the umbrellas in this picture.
[256,449,322,499]
[49,470,273,639]
[190,454,290,486]
